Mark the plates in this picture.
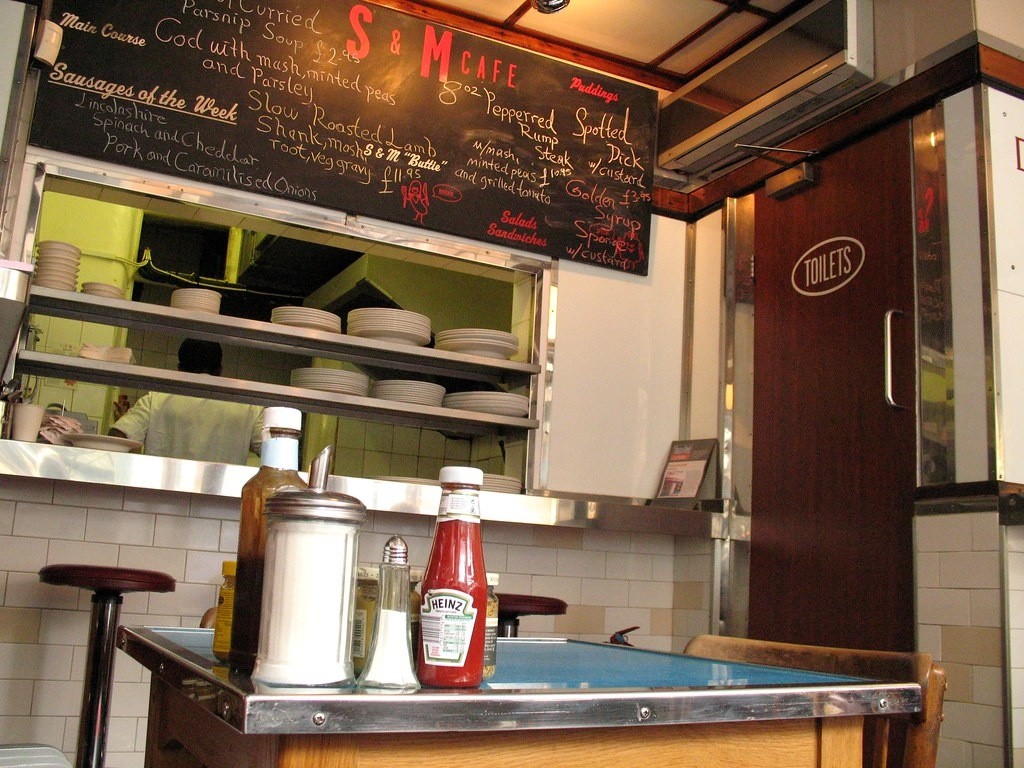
[290,367,371,397]
[347,308,432,347]
[442,391,529,418]
[270,306,342,334]
[32,240,81,292]
[434,327,519,360]
[80,282,125,300]
[369,379,446,407]
[480,473,522,494]
[170,288,222,315]
[60,433,144,453]
[78,342,136,365]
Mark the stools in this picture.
[498,593,568,637]
[38,563,177,768]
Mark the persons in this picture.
[107,338,267,466]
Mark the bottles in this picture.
[212,560,237,662]
[227,407,315,675]
[351,533,422,695]
[413,465,488,688]
[249,488,367,695]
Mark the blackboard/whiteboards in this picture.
[29,1,660,279]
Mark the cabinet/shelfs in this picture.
[18,283,542,437]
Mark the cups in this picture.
[12,403,45,442]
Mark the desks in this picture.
[117,624,921,768]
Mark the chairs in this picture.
[683,633,947,768]
